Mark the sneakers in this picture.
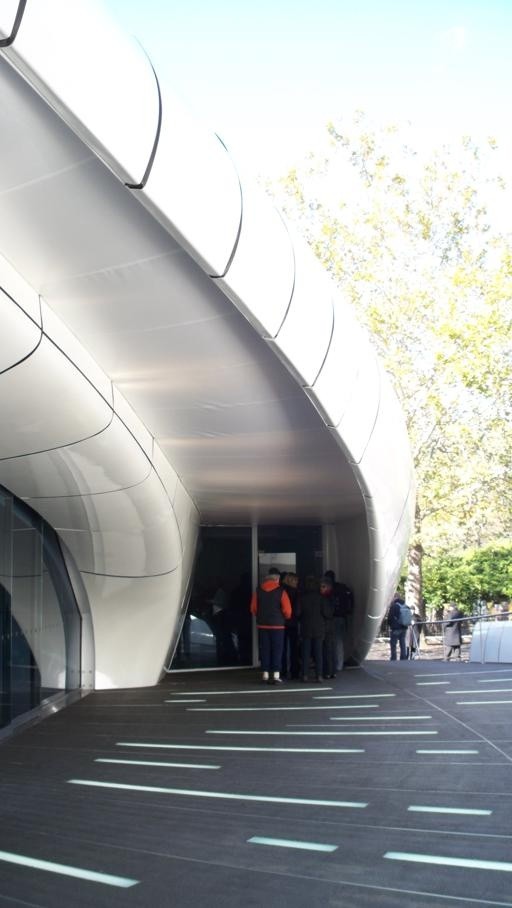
[258,678,288,686]
[302,673,337,684]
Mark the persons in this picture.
[251,568,355,683]
[175,615,193,662]
[386,590,411,661]
[410,606,422,647]
[404,626,417,660]
[444,611,464,662]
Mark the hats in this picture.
[267,567,281,575]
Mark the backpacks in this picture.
[393,602,412,626]
